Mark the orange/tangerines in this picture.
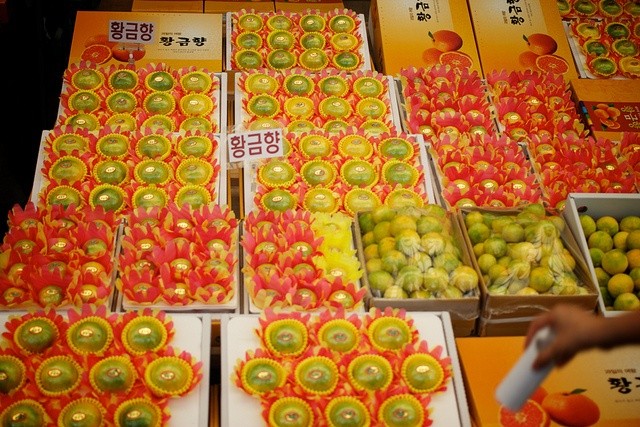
[244,214,355,305]
[244,318,445,427]
[581,214,639,310]
[243,71,390,139]
[233,13,360,72]
[49,68,210,209]
[492,80,583,139]
[465,203,585,295]
[533,142,639,196]
[2,217,114,304]
[358,202,480,301]
[127,217,231,300]
[404,76,488,144]
[437,143,536,205]
[555,0,639,83]
[258,135,429,213]
[0,317,189,426]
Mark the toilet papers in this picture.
[495,324,557,413]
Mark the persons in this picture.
[522,303,640,372]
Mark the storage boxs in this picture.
[394,79,500,135]
[54,71,228,134]
[525,135,640,203]
[220,310,473,426]
[0,219,124,312]
[562,20,640,81]
[226,10,372,70]
[241,217,366,317]
[66,11,223,75]
[467,0,580,100]
[454,334,640,427]
[238,132,436,221]
[367,0,484,86]
[115,218,242,347]
[350,206,482,337]
[275,0,345,13]
[457,205,600,337]
[204,0,275,38]
[562,190,640,321]
[426,139,546,212]
[566,77,640,146]
[0,311,212,427]
[28,128,232,221]
[234,71,403,135]
[481,79,591,141]
[131,0,203,13]
